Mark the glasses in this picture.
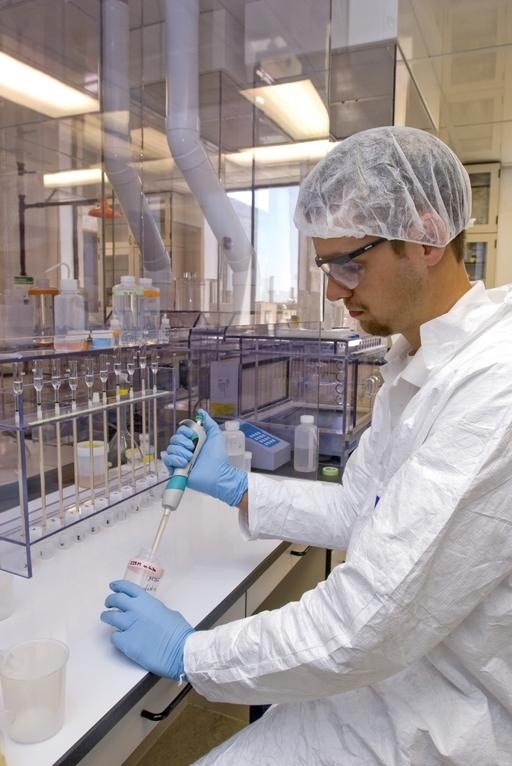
[314,238,385,290]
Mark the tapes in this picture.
[323,467,338,476]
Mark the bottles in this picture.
[222,419,246,468]
[106,544,166,636]
[136,277,161,333]
[53,279,86,351]
[111,274,144,341]
[108,405,140,466]
[293,414,319,472]
[74,439,111,489]
[8,274,36,336]
[25,276,61,334]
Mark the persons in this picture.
[98,123,512,766]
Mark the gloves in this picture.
[100,579,196,682]
[163,408,247,506]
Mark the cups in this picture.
[1,638,70,746]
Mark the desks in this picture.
[0,472,333,766]
